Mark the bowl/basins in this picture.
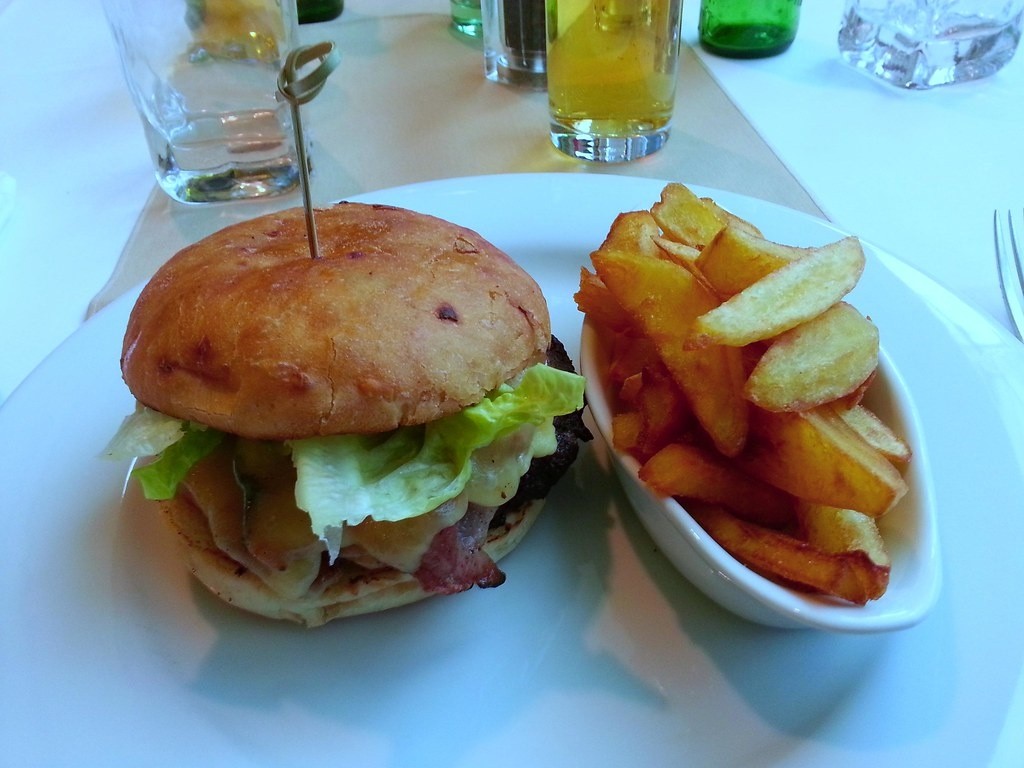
[580,303,940,632]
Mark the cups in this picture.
[545,0,683,163]
[100,0,310,205]
[449,0,547,91]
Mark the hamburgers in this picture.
[97,200,593,629]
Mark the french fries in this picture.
[571,180,912,612]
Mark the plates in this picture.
[0,174,1024,768]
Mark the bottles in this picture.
[700,0,802,58]
[296,0,344,25]
